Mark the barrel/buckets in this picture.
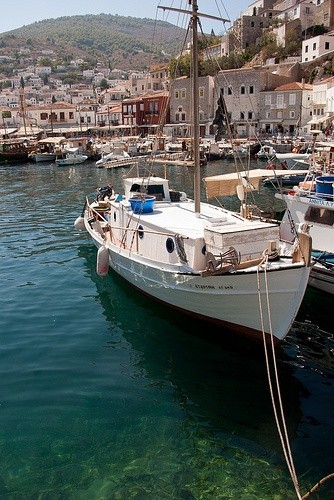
[315,176,334,201]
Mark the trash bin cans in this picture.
[315,175,334,201]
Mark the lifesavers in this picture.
[269,165,276,170]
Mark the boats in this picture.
[256,145,275,159]
[81,0,316,340]
[263,129,333,294]
[0,131,143,170]
[143,136,248,167]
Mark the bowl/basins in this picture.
[128,196,156,213]
